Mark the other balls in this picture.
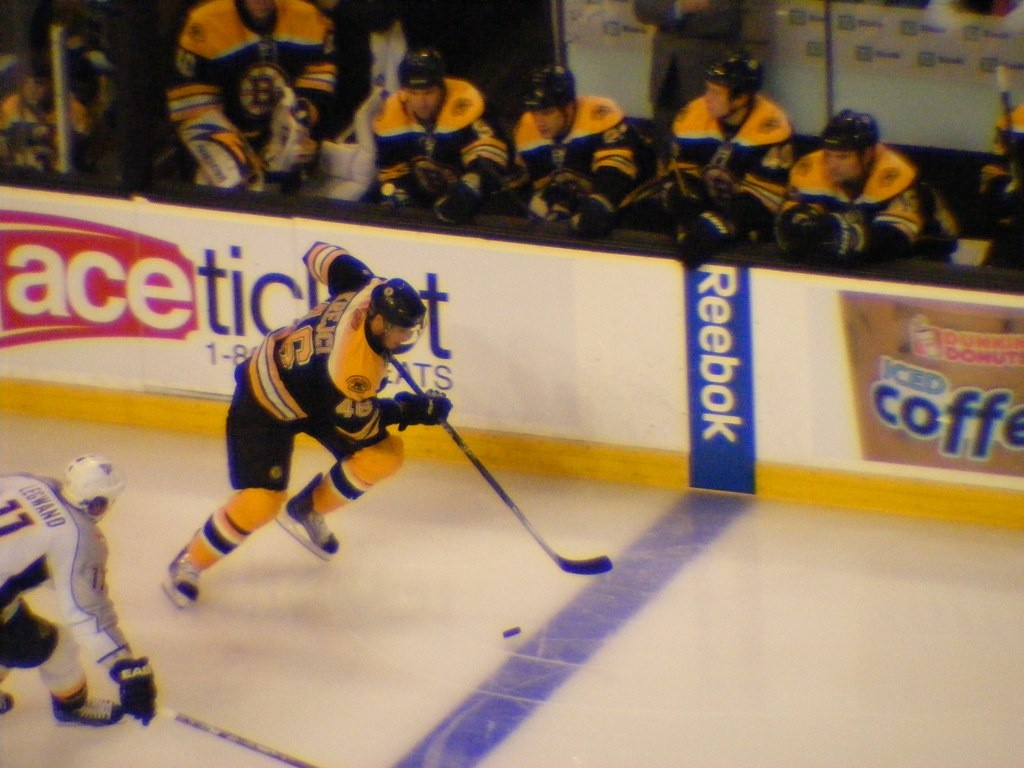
[503,626,521,637]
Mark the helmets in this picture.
[59,454,124,524]
[397,47,445,92]
[518,63,574,113]
[820,110,879,151]
[702,49,764,102]
[368,278,427,354]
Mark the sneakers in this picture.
[275,472,343,561]
[0,693,14,714]
[161,548,201,609]
[50,683,126,727]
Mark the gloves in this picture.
[430,179,483,227]
[109,655,158,727]
[775,200,852,268]
[654,163,749,270]
[565,194,617,242]
[394,388,453,431]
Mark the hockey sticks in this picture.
[388,353,614,575]
[154,704,316,767]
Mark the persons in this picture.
[167,241,453,600]
[166,1,336,196]
[978,103,1024,236]
[773,108,958,272]
[634,0,777,167]
[652,57,793,270]
[369,46,649,237]
[0,453,158,728]
[0,0,121,186]
